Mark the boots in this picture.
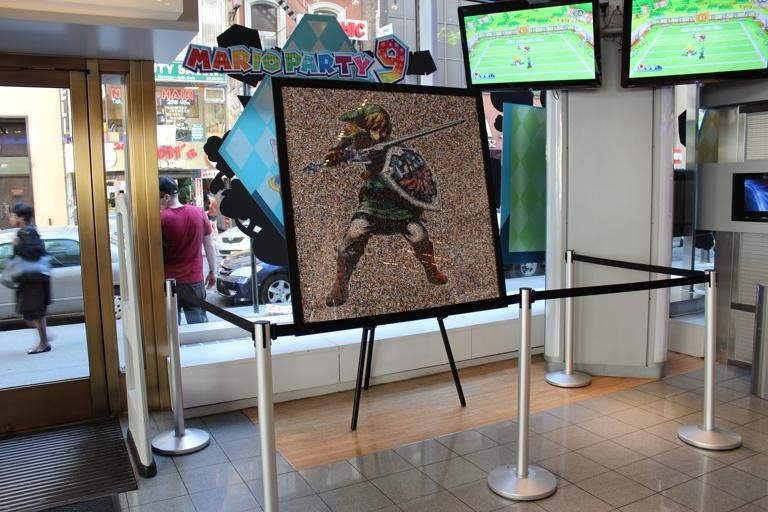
[414,242,448,284]
[326,256,359,306]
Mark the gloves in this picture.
[324,147,343,167]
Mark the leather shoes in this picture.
[28,345,51,354]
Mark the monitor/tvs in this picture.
[732,172,768,222]
[458,0,602,92]
[621,0,768,89]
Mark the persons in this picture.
[157,175,218,324]
[9,203,52,354]
[203,194,218,237]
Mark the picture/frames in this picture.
[270,74,507,337]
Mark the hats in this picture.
[339,104,387,123]
[159,176,178,195]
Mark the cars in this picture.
[0,226,128,319]
[216,219,292,304]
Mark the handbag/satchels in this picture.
[0,255,53,289]
